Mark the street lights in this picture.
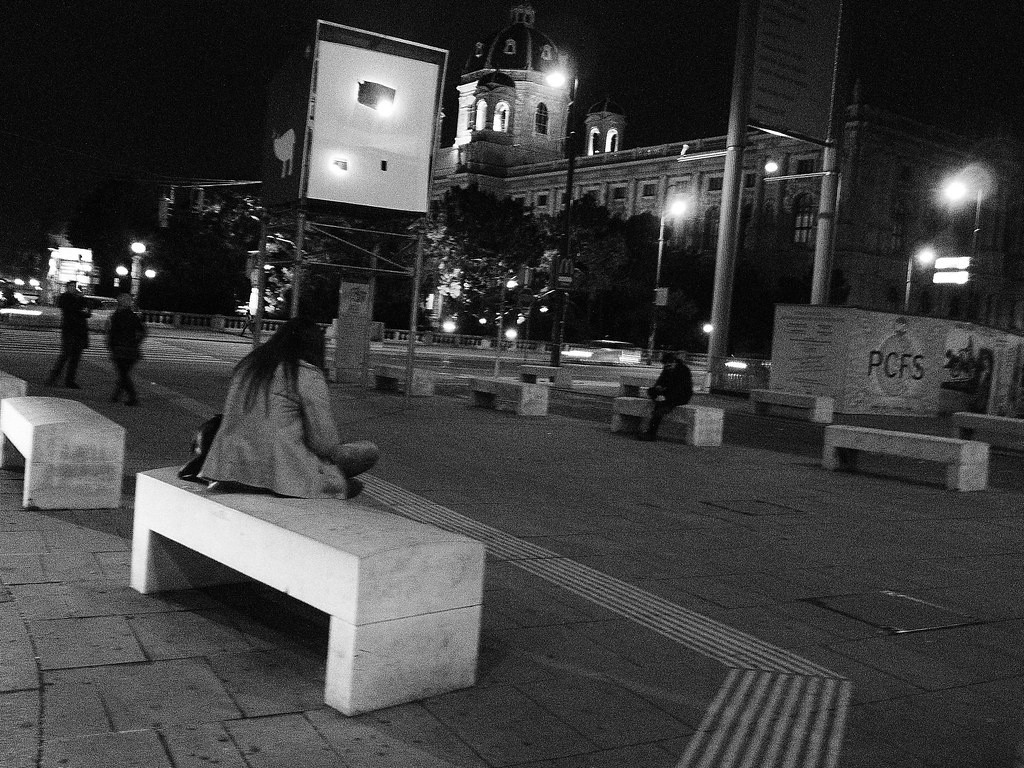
[904,181,968,314]
[116,242,157,305]
[648,195,688,361]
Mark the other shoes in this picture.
[123,399,138,406]
[654,394,666,402]
[63,380,79,389]
[107,394,119,403]
[45,380,59,388]
[636,432,657,442]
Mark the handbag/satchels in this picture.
[177,413,224,478]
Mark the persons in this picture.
[45,280,91,388]
[196,315,382,501]
[104,292,149,408]
[240,309,257,337]
[647,352,694,442]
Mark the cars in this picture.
[561,337,642,366]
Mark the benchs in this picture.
[0,397,126,510]
[130,468,485,715]
[821,424,990,492]
[375,364,437,396]
[953,412,1024,442]
[751,389,835,423]
[470,377,548,417]
[520,365,564,383]
[609,396,724,447]
[618,376,656,396]
